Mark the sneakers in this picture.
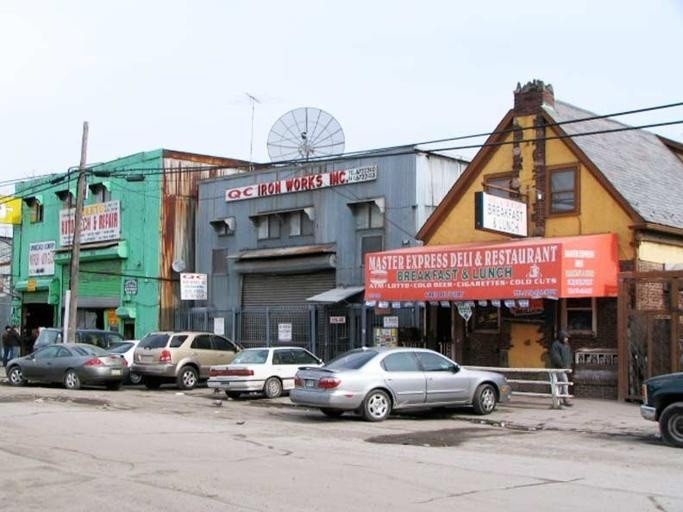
[564,399,573,405]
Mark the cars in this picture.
[640,371,683,448]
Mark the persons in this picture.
[549,329,575,407]
[2,325,11,367]
[8,324,23,360]
